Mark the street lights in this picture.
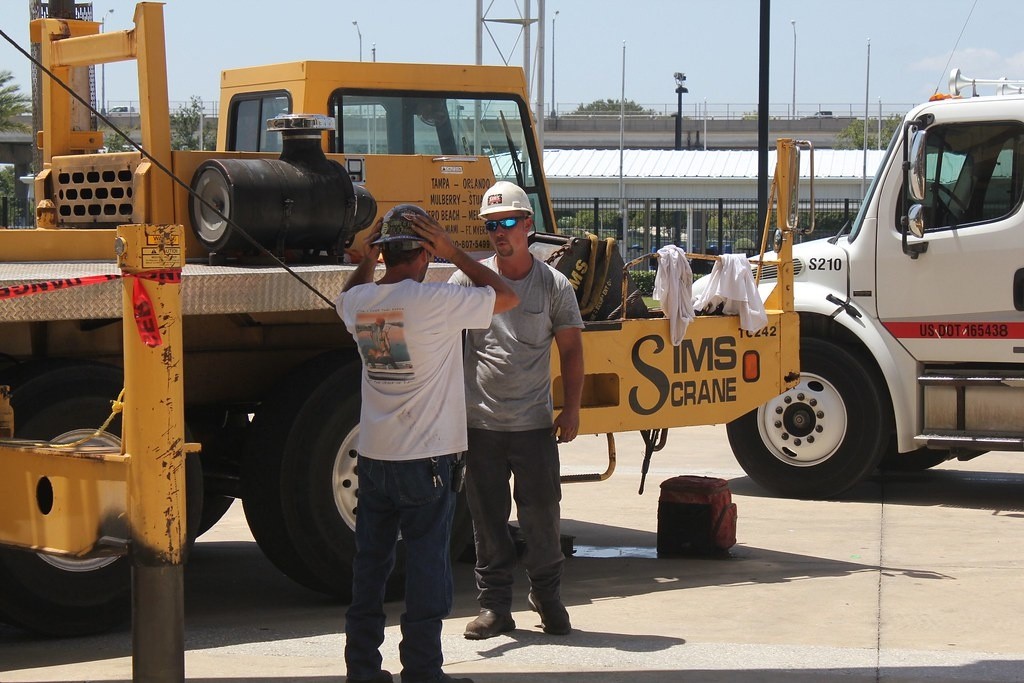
[552,10,559,116]
[101,9,114,114]
[790,19,796,119]
[352,20,362,116]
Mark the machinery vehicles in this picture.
[0,0,814,643]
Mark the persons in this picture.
[445,180,586,639]
[336,204,520,683]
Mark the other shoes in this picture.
[346,670,393,683]
[401,671,474,683]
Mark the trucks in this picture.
[691,68,1024,502]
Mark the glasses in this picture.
[484,216,528,232]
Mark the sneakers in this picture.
[528,589,571,635]
[464,608,516,639]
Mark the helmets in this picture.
[478,181,533,220]
[370,204,432,245]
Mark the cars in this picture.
[109,106,135,112]
